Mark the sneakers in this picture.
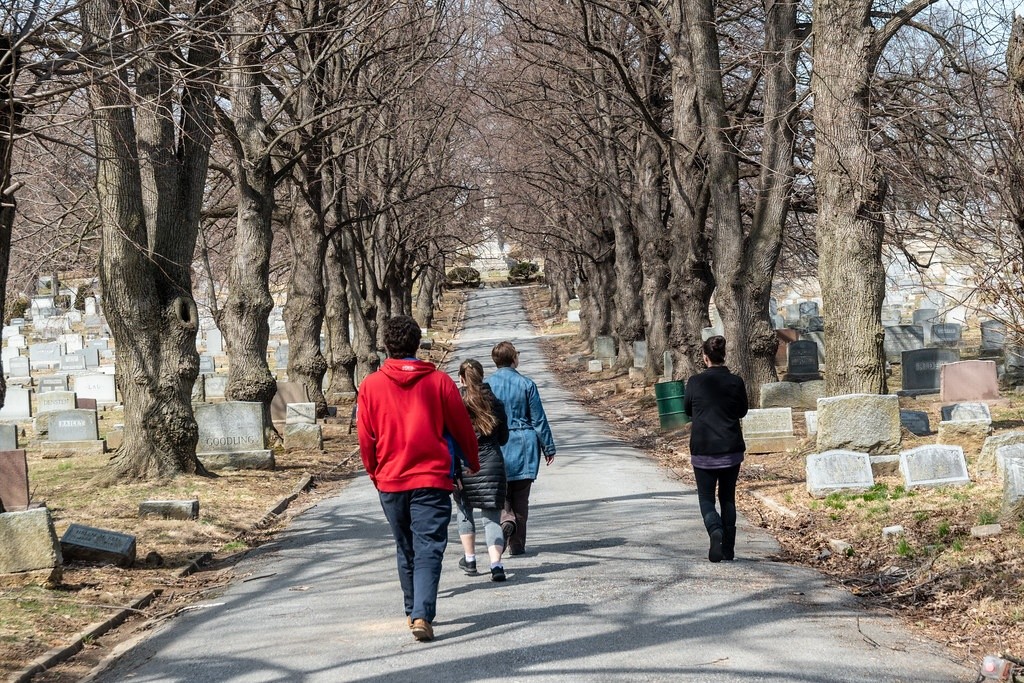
[459,554,477,572]
[490,566,506,581]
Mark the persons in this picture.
[685,336,748,563]
[358,316,481,641]
[483,341,556,555]
[453,358,509,580]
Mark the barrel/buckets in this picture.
[654,380,691,431]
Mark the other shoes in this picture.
[498,521,514,553]
[507,538,525,555]
[407,614,415,629]
[411,619,434,641]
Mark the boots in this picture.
[703,512,722,563]
[718,525,737,561]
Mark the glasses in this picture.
[515,351,520,356]
